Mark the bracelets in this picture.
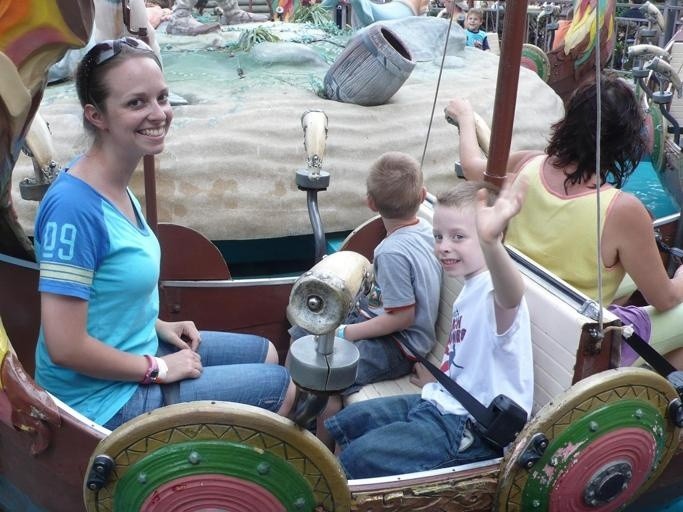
[140,352,158,385]
[337,323,347,338]
[154,356,168,385]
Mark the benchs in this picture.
[0,207,116,511]
[335,210,599,431]
[421,190,623,371]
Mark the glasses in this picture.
[83,36,153,103]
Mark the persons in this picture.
[324,170,535,484]
[442,73,682,381]
[165,1,268,35]
[621,0,646,20]
[464,6,492,54]
[436,0,468,29]
[314,151,443,456]
[31,36,298,433]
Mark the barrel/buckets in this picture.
[322,22,416,105]
